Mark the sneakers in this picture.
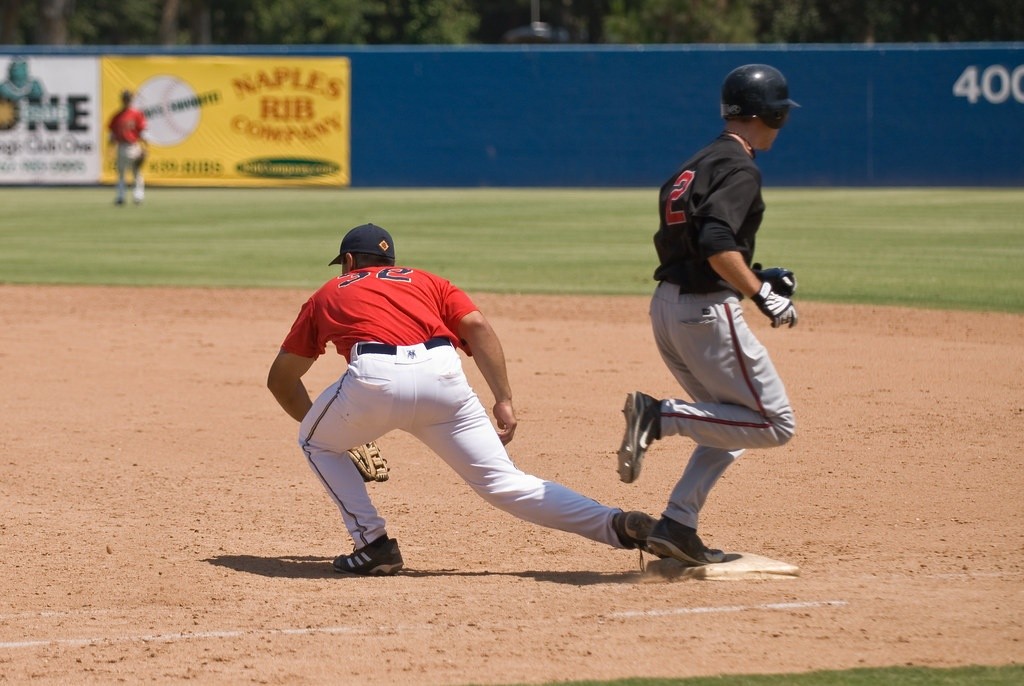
[646,514,726,566]
[612,509,670,572]
[616,390,660,484]
[332,534,404,576]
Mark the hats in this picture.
[328,223,395,266]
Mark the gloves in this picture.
[752,263,798,297]
[750,281,798,329]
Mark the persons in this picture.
[266,223,656,574]
[108,91,148,205]
[617,65,803,565]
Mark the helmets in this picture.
[720,63,801,120]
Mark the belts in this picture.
[658,283,726,295]
[357,337,451,355]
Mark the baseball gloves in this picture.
[134,150,147,168]
[345,439,392,483]
